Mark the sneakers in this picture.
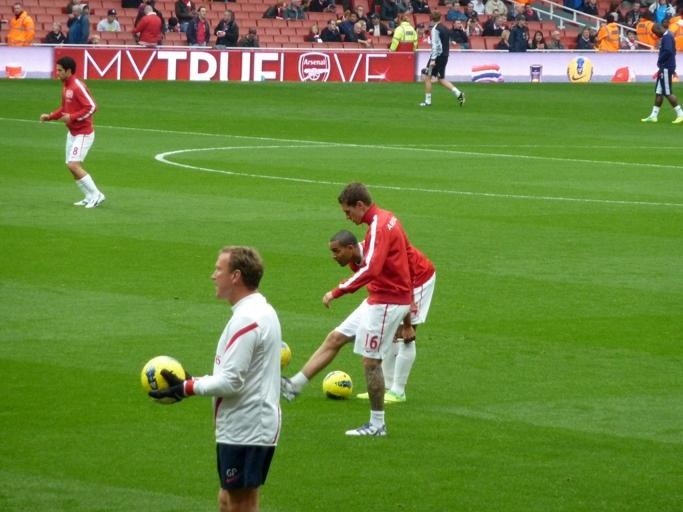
[457,92,465,107]
[419,101,431,106]
[84,191,105,209]
[345,423,387,437]
[356,388,390,400]
[641,116,658,122]
[281,376,298,401]
[383,390,406,404]
[73,198,99,207]
[672,117,683,123]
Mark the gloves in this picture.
[148,369,192,401]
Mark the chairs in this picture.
[1,0,652,51]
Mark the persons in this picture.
[641,23,683,123]
[39,58,105,208]
[326,228,434,405]
[548,0,683,49]
[146,245,282,510]
[281,182,412,437]
[263,0,548,52]
[419,13,465,108]
[7,2,258,49]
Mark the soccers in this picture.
[322,371,354,399]
[280,341,292,369]
[141,356,185,393]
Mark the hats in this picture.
[108,9,116,15]
[371,13,380,18]
